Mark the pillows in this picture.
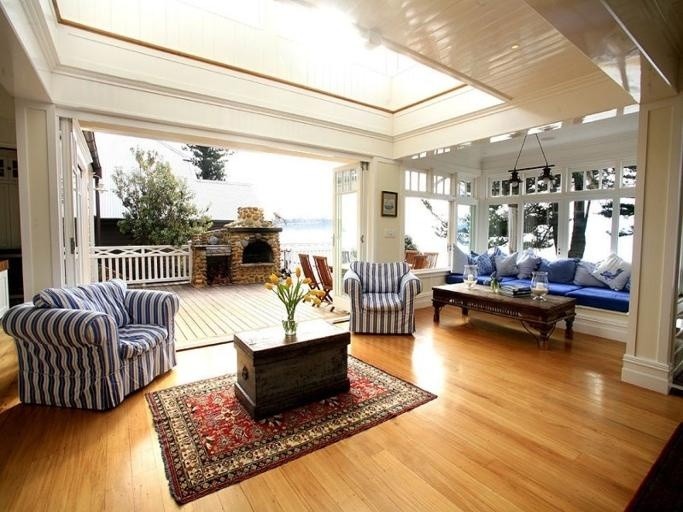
[449,244,629,292]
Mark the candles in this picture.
[536,282,544,289]
[467,275,473,281]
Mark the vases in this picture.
[281,315,297,336]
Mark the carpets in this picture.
[141,353,438,508]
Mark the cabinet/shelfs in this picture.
[0,142,21,251]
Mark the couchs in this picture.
[443,273,629,346]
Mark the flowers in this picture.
[262,264,326,317]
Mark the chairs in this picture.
[412,255,427,270]
[296,251,322,303]
[340,259,423,342]
[312,254,332,313]
[0,276,179,413]
[422,252,439,269]
[405,250,419,264]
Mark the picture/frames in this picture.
[380,189,398,219]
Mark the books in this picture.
[499,285,531,298]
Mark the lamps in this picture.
[501,133,559,186]
